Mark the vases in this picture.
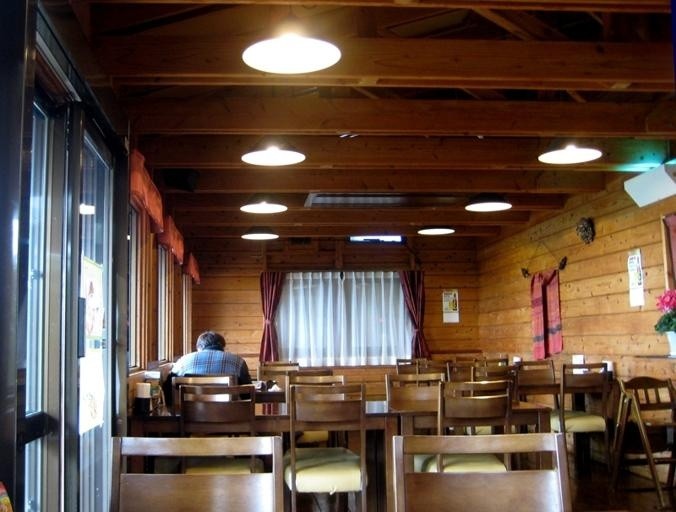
[664,330,676,357]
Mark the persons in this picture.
[170,330,252,401]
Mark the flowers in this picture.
[653,288,676,332]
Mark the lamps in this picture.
[416,137,602,235]
[237,6,342,241]
